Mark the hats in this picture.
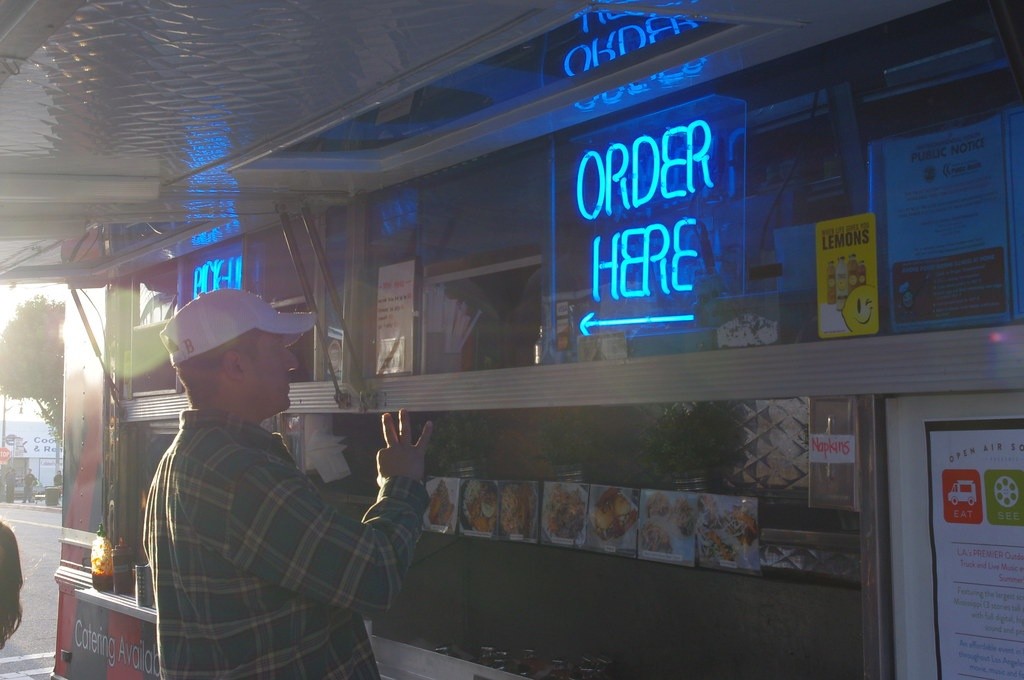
[158,290,319,369]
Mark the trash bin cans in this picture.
[44,485,61,506]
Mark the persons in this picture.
[142,288,434,680]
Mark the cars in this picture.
[4,476,45,498]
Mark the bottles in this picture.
[112,538,132,594]
[91,523,115,591]
[535,325,545,365]
[826,254,865,312]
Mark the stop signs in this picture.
[0,447,11,463]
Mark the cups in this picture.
[135,564,153,608]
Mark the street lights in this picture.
[1,402,24,502]
